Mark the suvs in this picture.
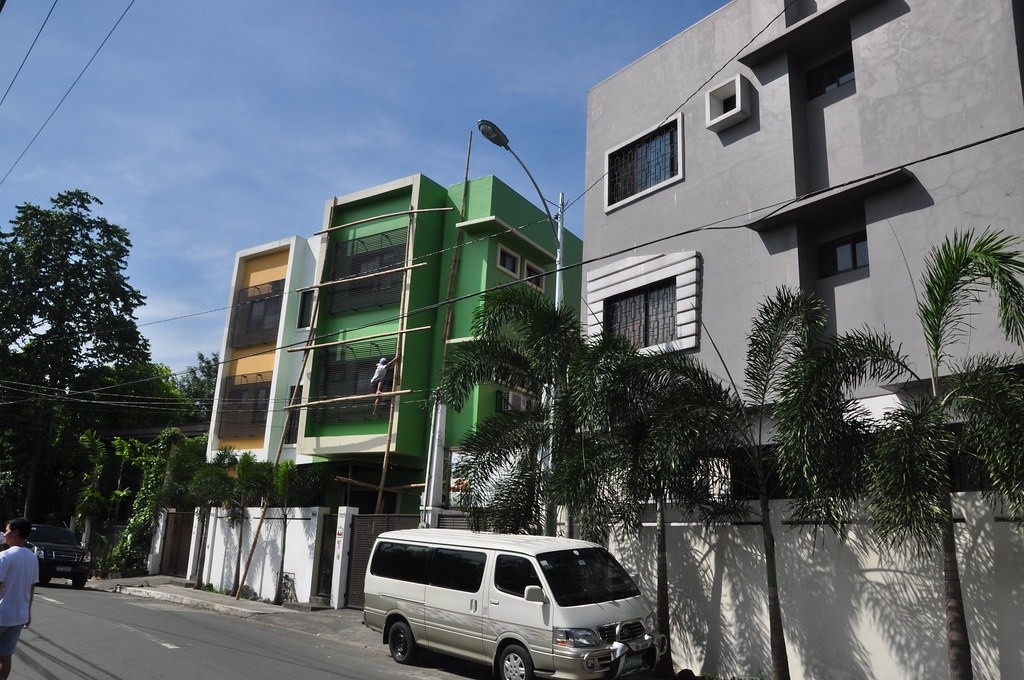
[20,524,94,589]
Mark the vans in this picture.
[362,527,667,680]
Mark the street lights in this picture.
[477,118,573,540]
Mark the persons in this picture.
[370,356,399,415]
[0,519,39,680]
